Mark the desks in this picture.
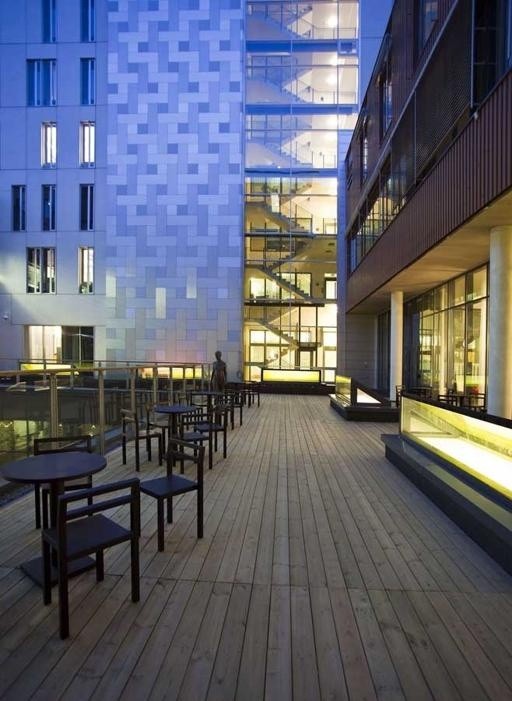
[1,452,108,589]
[154,406,196,466]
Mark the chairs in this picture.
[41,477,141,641]
[34,434,93,532]
[170,413,213,474]
[134,437,207,552]
[194,405,228,471]
[177,382,262,431]
[121,409,161,473]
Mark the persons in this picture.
[210,350,228,405]
